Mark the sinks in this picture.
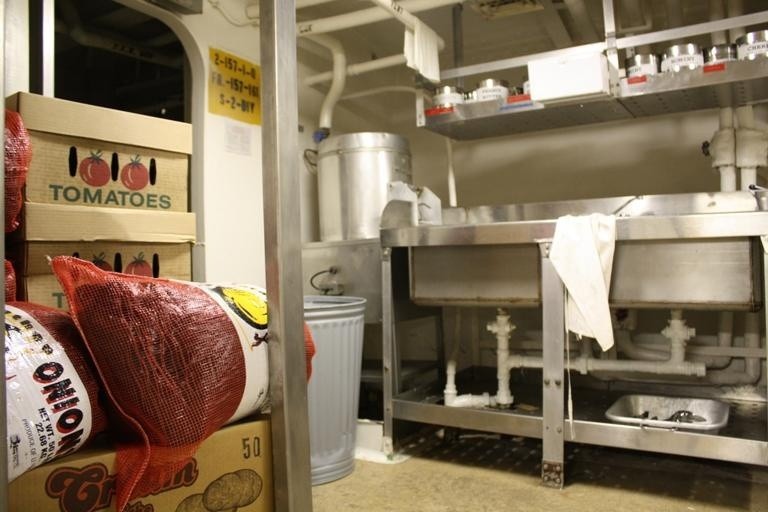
[405,220,554,306]
[609,213,763,312]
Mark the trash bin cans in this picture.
[299,294,368,486]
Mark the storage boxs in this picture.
[19,200,196,310]
[5,91,193,211]
[7,412,275,511]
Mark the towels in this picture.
[403,17,441,84]
[548,212,617,353]
